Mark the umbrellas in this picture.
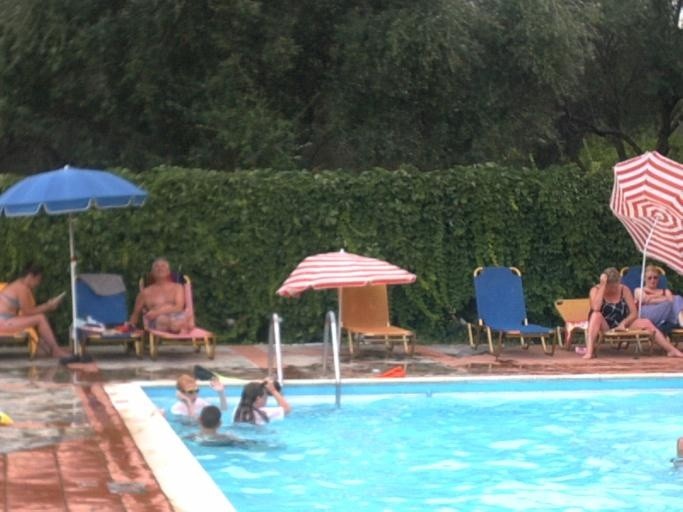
[275,249,417,346]
[608,151,683,354]
[0,165,147,364]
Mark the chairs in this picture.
[554,298,657,356]
[467,266,557,357]
[337,284,417,357]
[138,274,220,361]
[68,272,145,361]
[0,282,39,360]
[619,266,683,347]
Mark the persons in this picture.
[0,262,73,359]
[634,263,683,328]
[128,256,191,333]
[233,378,291,425]
[582,267,683,360]
[171,374,226,415]
[183,406,265,447]
